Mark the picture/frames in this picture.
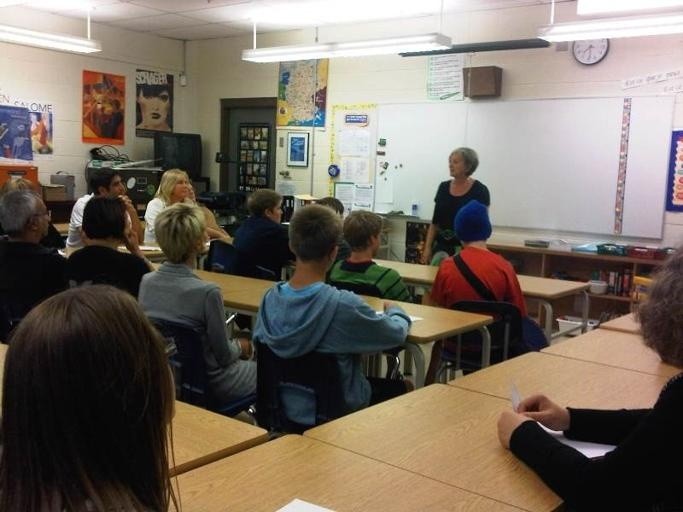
[286,131,310,167]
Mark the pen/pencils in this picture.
[225,312,238,324]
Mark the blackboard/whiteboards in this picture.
[329,95,673,240]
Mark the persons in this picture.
[495,243,683,511]
[137,204,256,400]
[2,179,68,345]
[419,147,491,306]
[232,188,296,277]
[422,200,528,386]
[254,204,415,430]
[101,100,123,138]
[136,84,172,132]
[1,282,182,512]
[65,167,225,297]
[318,197,414,302]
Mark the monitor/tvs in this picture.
[154,131,202,178]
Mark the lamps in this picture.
[0,7,104,56]
[536,1,683,43]
[242,0,452,64]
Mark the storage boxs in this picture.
[44,185,64,201]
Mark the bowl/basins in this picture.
[589,279,608,295]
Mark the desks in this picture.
[0,223,683,512]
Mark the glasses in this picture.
[33,210,51,216]
[163,335,178,358]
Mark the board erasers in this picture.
[525,240,549,248]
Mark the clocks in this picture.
[572,39,609,66]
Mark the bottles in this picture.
[411,201,418,216]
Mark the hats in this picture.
[454,199,491,241]
